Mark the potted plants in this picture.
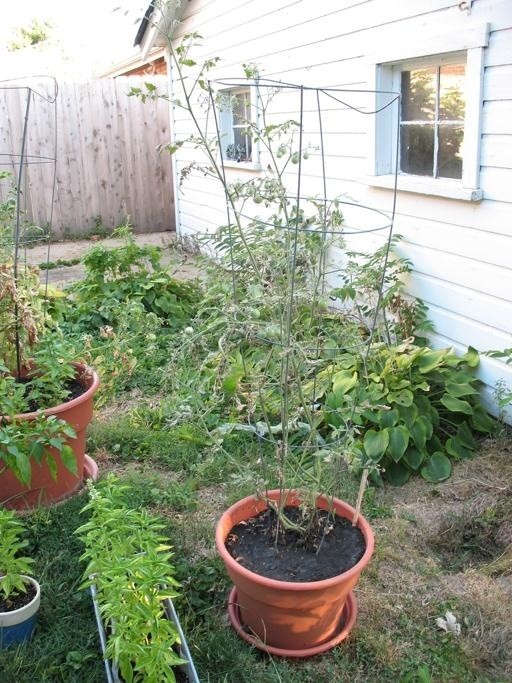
[73,471,202,683]
[0,152,100,513]
[116,0,380,659]
[0,351,82,644]
